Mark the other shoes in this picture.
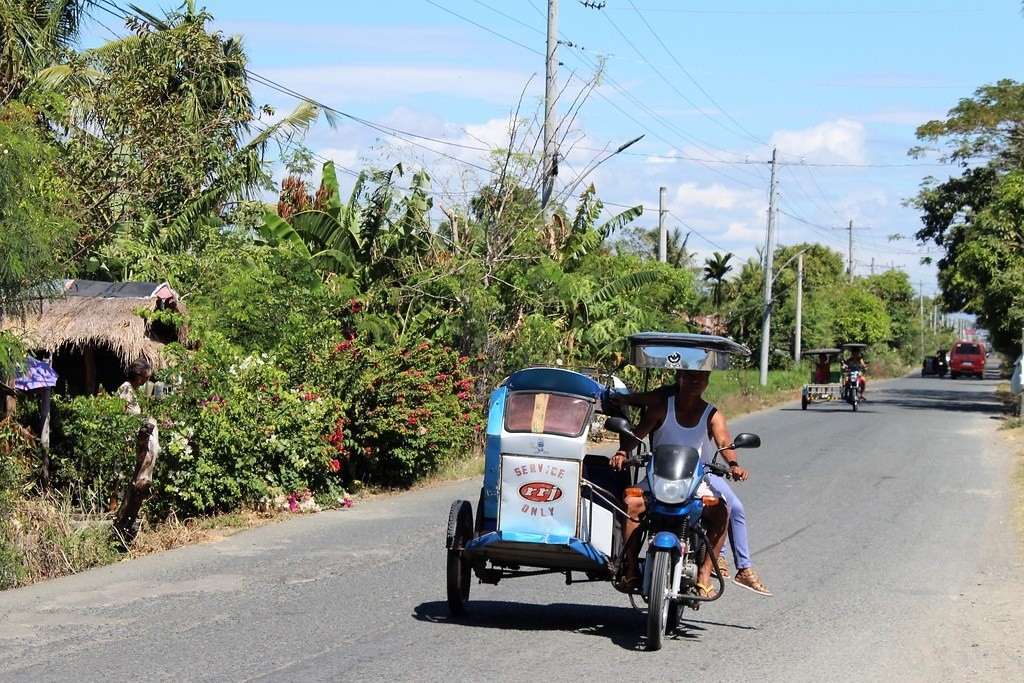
[861,395,867,400]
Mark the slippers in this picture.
[696,583,717,603]
[618,569,641,590]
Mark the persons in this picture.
[607,366,774,599]
[113,357,152,453]
[840,347,867,402]
[811,354,838,384]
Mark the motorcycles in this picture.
[800,343,867,411]
[920,349,950,379]
[442,332,749,652]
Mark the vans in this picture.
[949,339,986,380]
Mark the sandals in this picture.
[711,555,731,579]
[732,569,773,597]
